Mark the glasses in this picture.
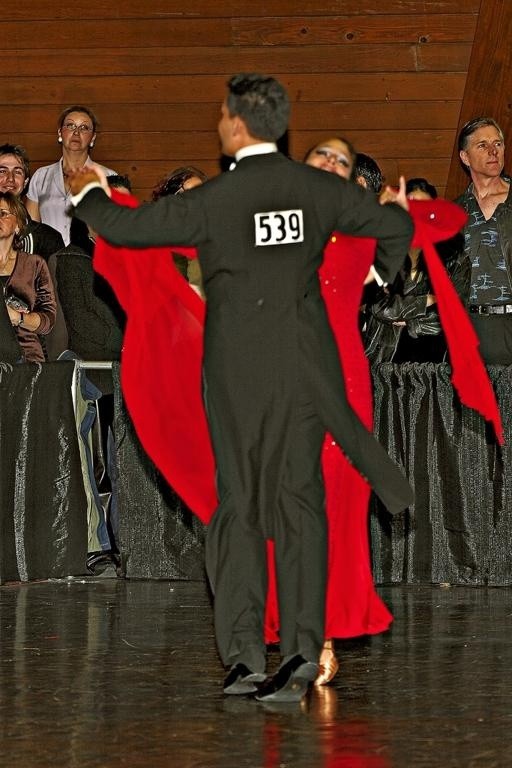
[0,211,13,218]
[62,123,93,132]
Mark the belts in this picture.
[469,304,512,317]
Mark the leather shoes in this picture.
[223,640,339,702]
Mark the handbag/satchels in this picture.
[4,295,29,314]
